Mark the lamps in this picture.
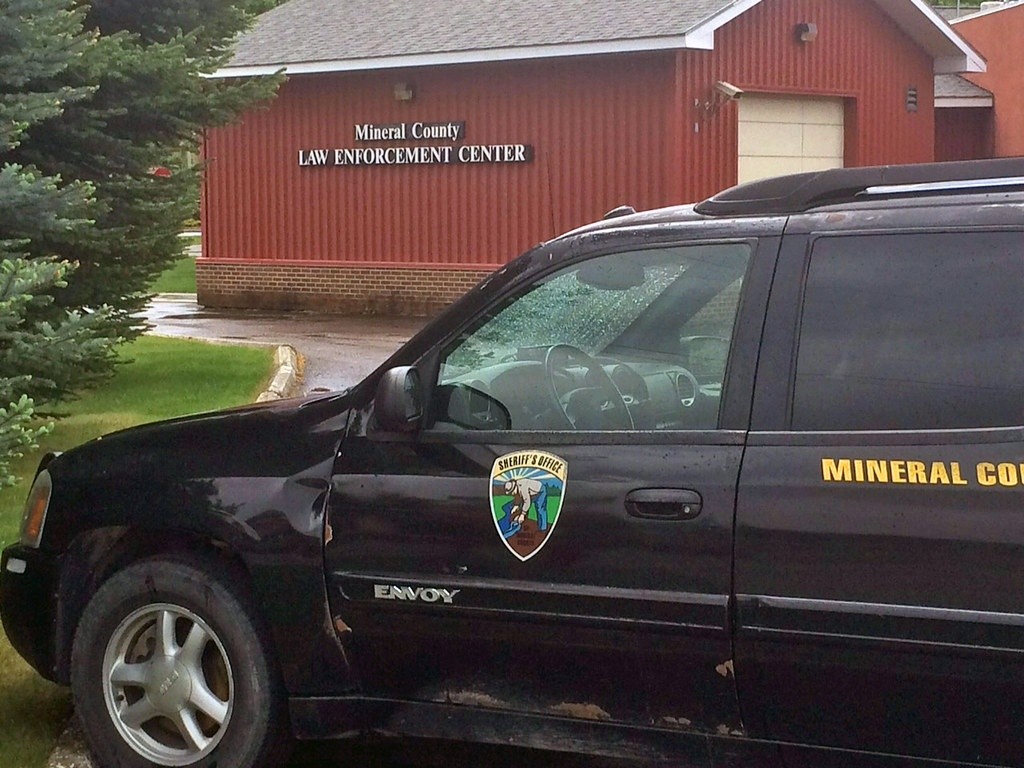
[394,84,414,99]
[798,23,817,44]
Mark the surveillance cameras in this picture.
[715,81,744,100]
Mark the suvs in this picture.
[0,148,1024,768]
